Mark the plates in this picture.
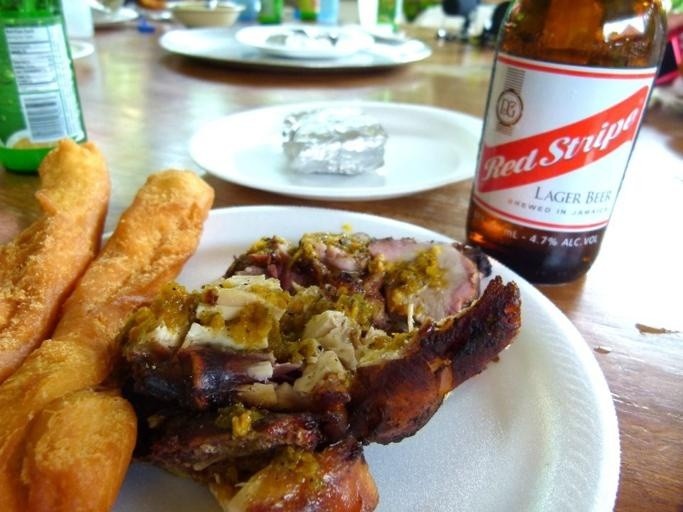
[103,205,621,512]
[235,25,375,60]
[191,101,483,201]
[158,25,432,69]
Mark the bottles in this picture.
[465,0,668,285]
[0,0,87,175]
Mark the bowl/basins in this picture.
[171,4,238,28]
